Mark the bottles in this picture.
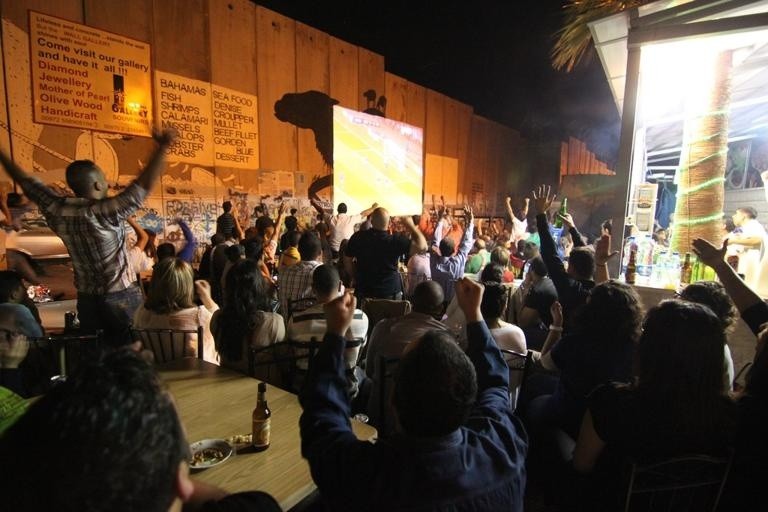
[250,381,272,450]
[621,234,715,292]
[551,197,568,231]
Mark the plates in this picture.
[189,439,232,471]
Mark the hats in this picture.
[0,302,44,338]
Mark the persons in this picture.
[1,125,180,339]
[287,182,767,512]
[1,192,287,511]
